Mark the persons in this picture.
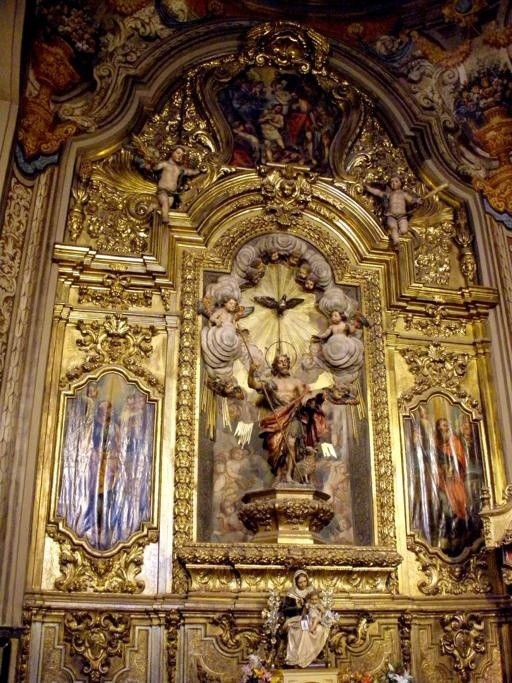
[361,175,424,248]
[220,74,331,164]
[146,145,207,223]
[209,297,356,339]
[247,353,327,482]
[67,391,151,537]
[410,414,471,549]
[278,569,327,663]
